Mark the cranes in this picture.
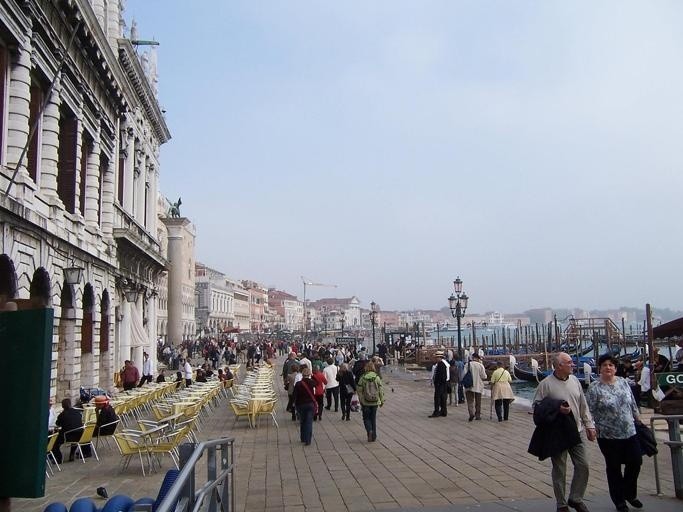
[300,275,340,318]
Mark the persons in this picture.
[75,395,118,458]
[427,350,465,417]
[463,353,488,422]
[675,339,683,371]
[585,354,658,511]
[651,345,669,371]
[395,338,423,352]
[121,360,139,391]
[155,338,386,445]
[490,362,512,422]
[137,352,153,386]
[631,361,651,405]
[47,397,56,446]
[119,359,134,377]
[52,399,82,463]
[530,353,596,511]
[618,359,635,379]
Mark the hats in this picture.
[92,395,110,404]
[633,361,644,369]
[302,368,312,376]
[433,351,444,357]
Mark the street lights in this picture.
[368,301,378,355]
[338,310,346,336]
[320,310,329,336]
[447,275,469,354]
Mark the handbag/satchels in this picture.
[448,384,451,394]
[462,372,473,388]
[345,384,355,394]
[314,400,319,414]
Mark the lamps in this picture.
[11,226,84,285]
[114,275,160,303]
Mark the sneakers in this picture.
[567,498,588,512]
[76,451,91,458]
[617,504,629,512]
[629,499,642,508]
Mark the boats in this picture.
[420,303,670,410]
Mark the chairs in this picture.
[45,361,279,480]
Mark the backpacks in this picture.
[365,379,379,402]
